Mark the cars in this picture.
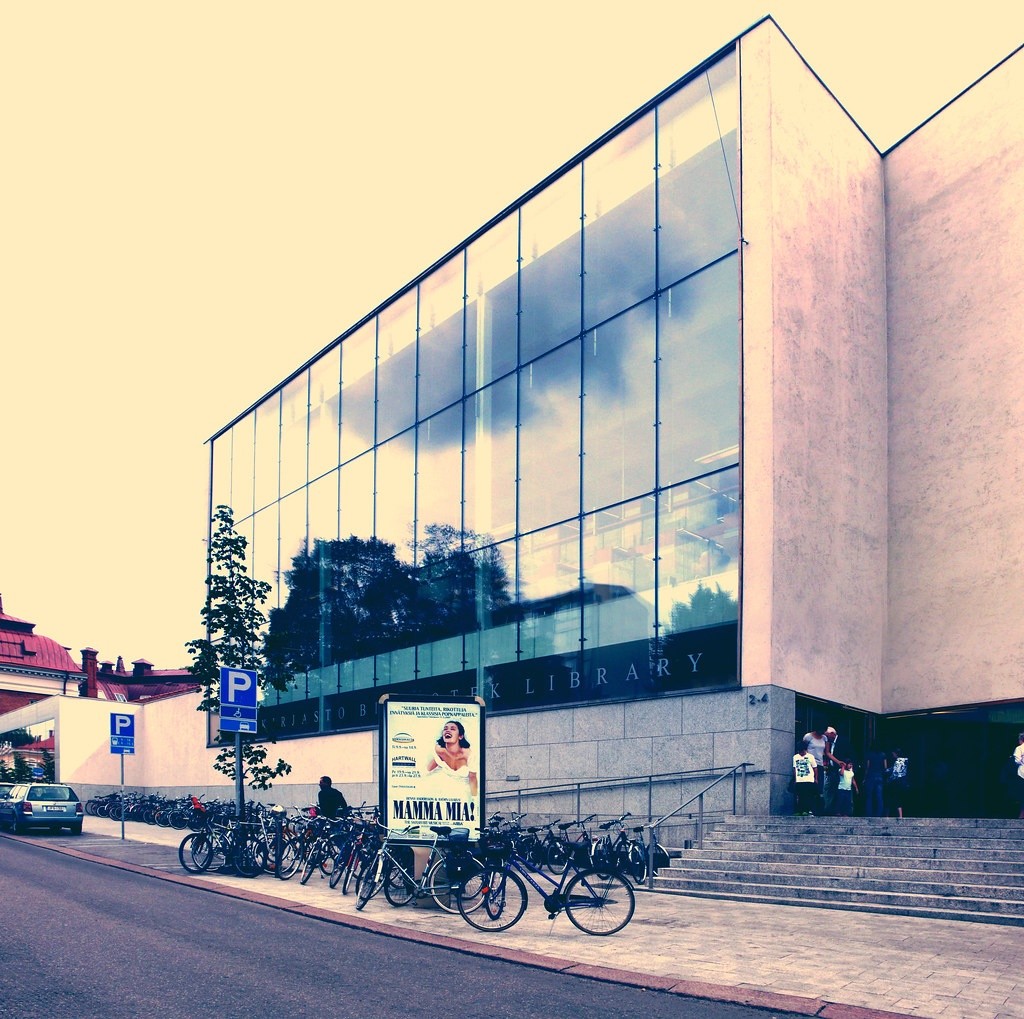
[1,783,14,808]
[0,783,84,835]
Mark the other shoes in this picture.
[802,809,808,816]
[792,813,801,816]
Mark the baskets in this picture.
[449,827,471,846]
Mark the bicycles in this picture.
[84,790,670,938]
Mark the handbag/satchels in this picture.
[887,771,898,785]
[445,851,473,878]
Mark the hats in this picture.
[825,726,836,735]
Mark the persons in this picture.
[792,721,908,818]
[1013,732,1024,819]
[426,721,478,798]
[318,776,348,868]
